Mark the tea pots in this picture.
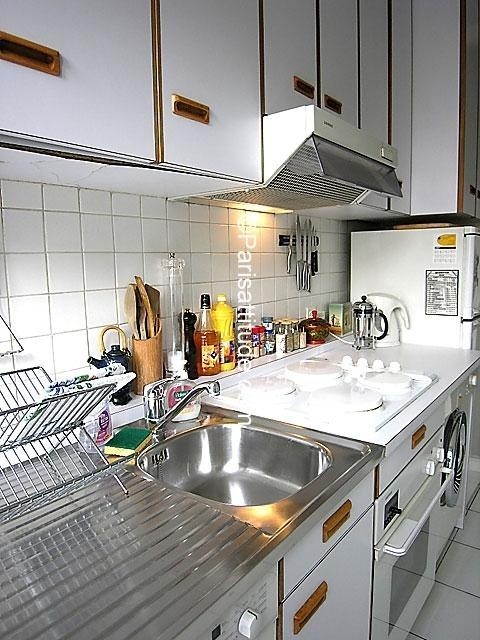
[85,325,135,406]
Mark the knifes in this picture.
[283,212,320,295]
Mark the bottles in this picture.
[158,249,248,380]
[298,309,332,345]
[274,318,308,355]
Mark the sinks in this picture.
[138,416,335,508]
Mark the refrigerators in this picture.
[346,223,479,351]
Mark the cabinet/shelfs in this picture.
[151,0,265,199]
[260,0,410,222]
[391,1,480,223]
[281,466,378,640]
[0,2,159,197]
[1,366,141,527]
[375,400,447,496]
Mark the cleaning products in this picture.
[161,352,201,422]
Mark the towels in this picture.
[7,362,138,440]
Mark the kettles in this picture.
[350,291,411,352]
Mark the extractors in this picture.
[164,101,406,217]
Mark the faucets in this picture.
[148,378,221,443]
